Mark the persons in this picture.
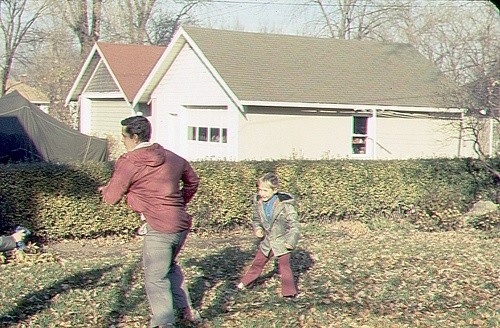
[96,115,201,328]
[237,172,302,299]
[0,225,26,254]
